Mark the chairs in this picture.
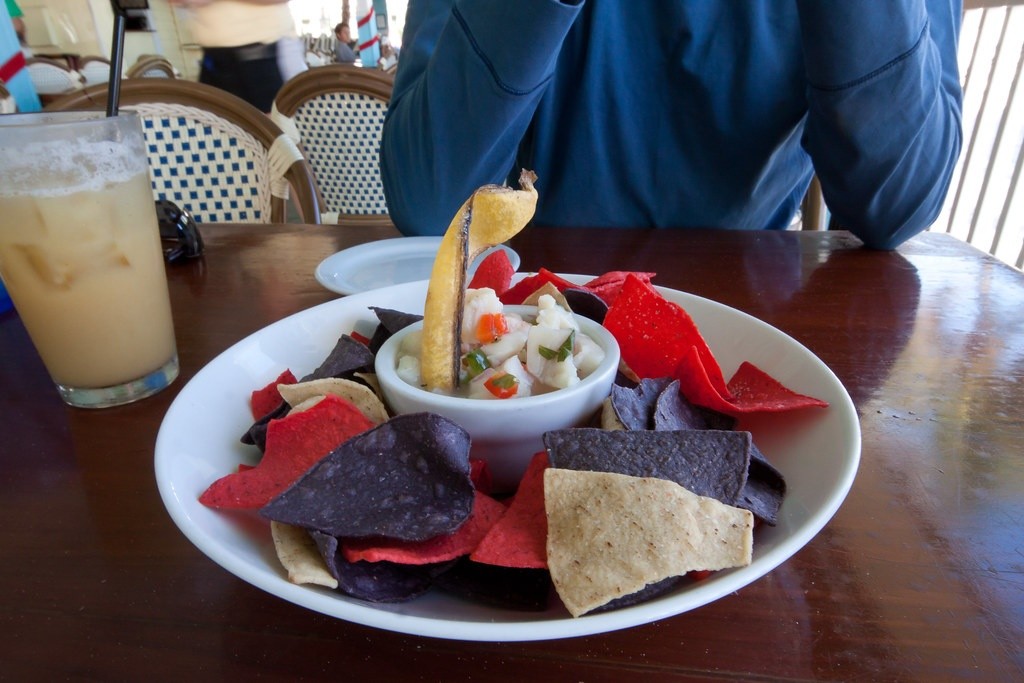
[272,65,396,227]
[304,47,337,68]
[41,77,323,224]
[26,54,184,94]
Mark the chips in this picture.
[197,251,832,621]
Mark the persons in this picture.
[5,0,27,35]
[169,0,301,113]
[335,24,355,63]
[380,0,963,253]
[379,36,400,60]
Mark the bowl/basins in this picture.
[374,305,621,471]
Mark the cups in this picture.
[0,110,180,409]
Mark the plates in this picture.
[314,237,521,296]
[153,271,862,642]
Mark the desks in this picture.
[0,224,1023,683]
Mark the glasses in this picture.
[155,200,204,264]
